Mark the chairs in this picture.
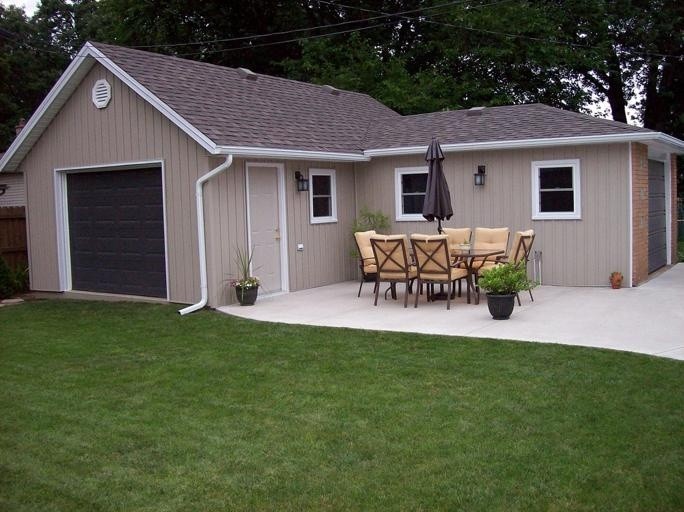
[472,227,513,275]
[353,229,397,299]
[409,232,472,310]
[369,234,416,308]
[440,225,473,297]
[477,228,536,307]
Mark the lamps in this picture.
[295,171,309,191]
[474,166,487,185]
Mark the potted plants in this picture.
[475,257,536,320]
[348,205,392,282]
[218,243,268,306]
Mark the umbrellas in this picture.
[420,136,453,235]
